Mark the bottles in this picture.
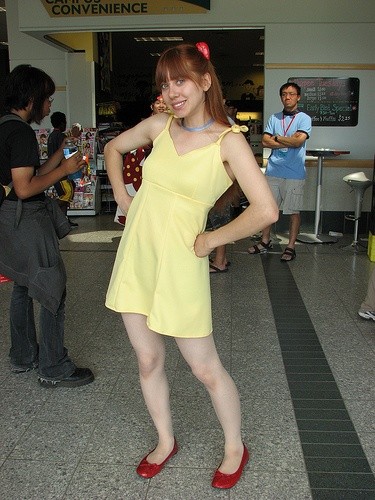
[63,139,82,179]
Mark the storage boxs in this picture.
[366,231,375,261]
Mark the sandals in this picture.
[281,247,296,263]
[247,239,273,254]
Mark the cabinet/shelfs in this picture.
[35,127,101,215]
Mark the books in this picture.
[34,128,55,160]
[64,128,121,210]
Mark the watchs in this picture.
[274,134,279,141]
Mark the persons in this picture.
[112,90,170,227]
[246,82,311,261]
[84,143,93,159]
[208,99,251,273]
[102,43,281,491]
[40,133,47,145]
[85,131,92,141]
[0,63,95,387]
[90,128,98,135]
[41,151,49,159]
[47,111,79,227]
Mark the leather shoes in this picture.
[39,367,95,389]
[11,343,67,374]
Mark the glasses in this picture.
[281,93,297,97]
[47,95,54,104]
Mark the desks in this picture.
[296,149,350,244]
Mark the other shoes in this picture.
[136,434,179,479]
[211,444,249,489]
[65,217,78,227]
[357,310,375,321]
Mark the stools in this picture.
[342,172,372,256]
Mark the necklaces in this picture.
[180,117,213,131]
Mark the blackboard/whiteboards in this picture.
[287,76,360,127]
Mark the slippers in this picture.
[209,258,231,266]
[209,264,228,273]
